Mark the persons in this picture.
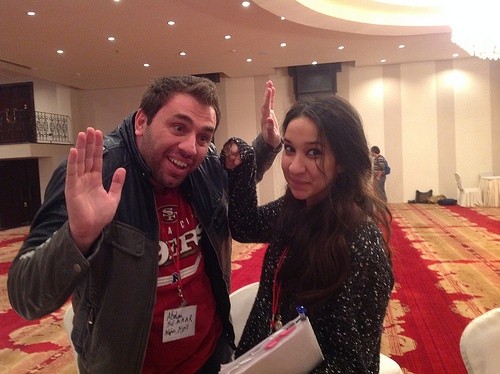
[6,74,285,374]
[370,146,387,203]
[220,93,395,374]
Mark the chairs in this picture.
[454,173,482,207]
[229,280,405,374]
[460,308,499,373]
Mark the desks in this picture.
[480,178,497,207]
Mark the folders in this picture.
[220,314,325,374]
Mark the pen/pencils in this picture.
[297,304,305,320]
